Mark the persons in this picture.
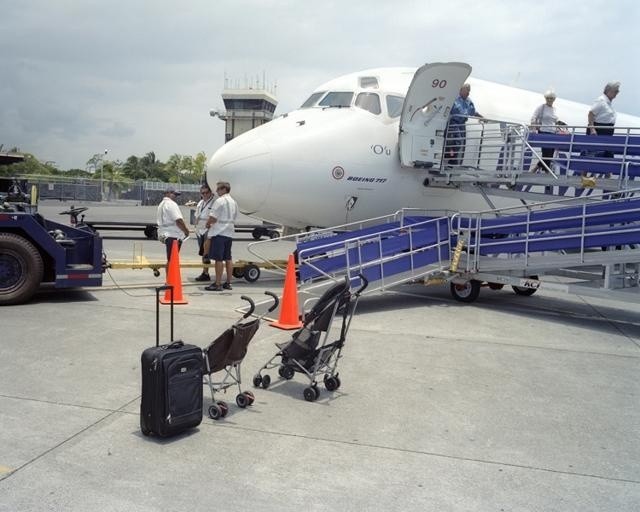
[530,90,565,195]
[156,187,189,286]
[580,81,621,199]
[194,184,218,282]
[205,180,238,291]
[444,83,487,167]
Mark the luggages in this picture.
[140,285,203,438]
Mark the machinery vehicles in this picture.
[0,152,107,300]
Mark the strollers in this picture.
[201,290,267,418]
[255,272,369,403]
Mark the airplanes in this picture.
[198,63,640,301]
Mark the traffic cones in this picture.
[159,240,190,304]
[267,254,304,330]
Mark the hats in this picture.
[165,187,181,195]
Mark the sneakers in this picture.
[196,272,210,281]
[205,282,223,291]
[223,282,233,290]
